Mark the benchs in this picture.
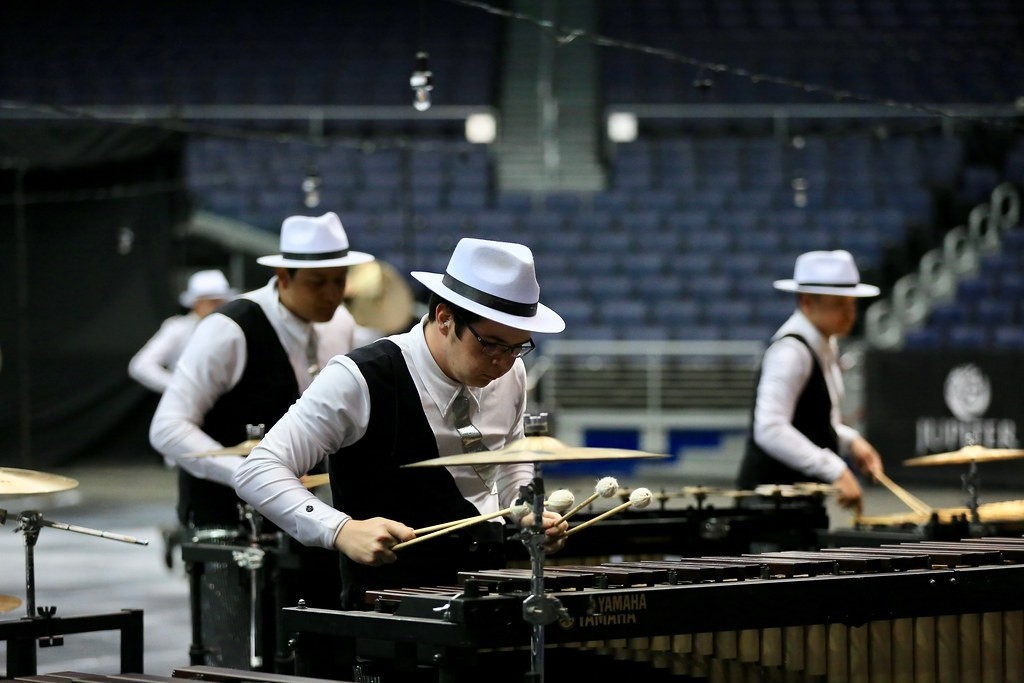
[545,341,764,453]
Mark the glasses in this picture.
[462,321,536,359]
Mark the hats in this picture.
[255,212,375,269]
[177,269,242,309]
[773,249,882,298]
[410,237,566,334]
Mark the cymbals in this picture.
[608,481,843,503]
[902,443,1024,467]
[398,436,675,470]
[0,466,80,496]
[180,439,265,460]
[0,592,22,613]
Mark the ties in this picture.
[307,327,321,383]
[452,389,499,495]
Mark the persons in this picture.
[229,238,569,614]
[150,211,377,667]
[127,269,234,569]
[738,250,883,526]
